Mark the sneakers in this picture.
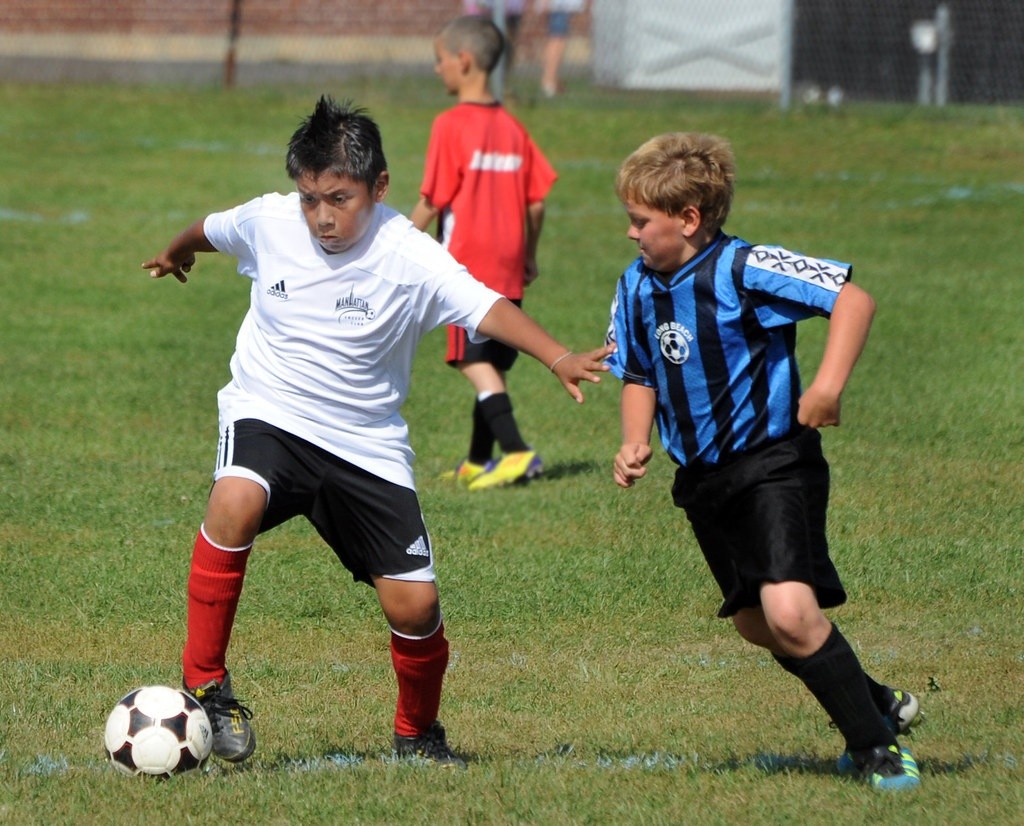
[440,456,494,486]
[838,694,922,776]
[470,452,545,491]
[392,722,471,774]
[866,749,921,793]
[179,669,258,763]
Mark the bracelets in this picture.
[550,350,576,374]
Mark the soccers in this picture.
[102,684,214,785]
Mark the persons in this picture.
[406,12,561,491]
[140,91,617,773]
[600,129,924,799]
[460,0,534,103]
[533,0,591,97]
